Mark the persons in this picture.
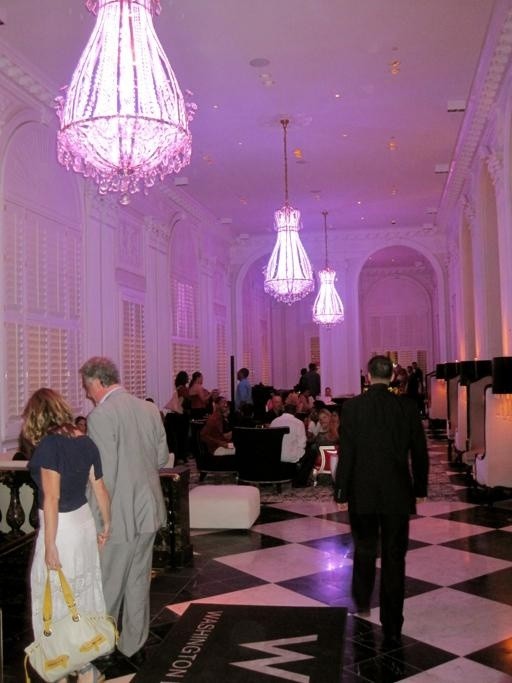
[144,355,425,490]
[332,356,430,639]
[77,355,169,671]
[74,417,90,433]
[21,387,111,683]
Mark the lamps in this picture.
[311,209,346,330]
[261,118,315,306]
[51,0,199,207]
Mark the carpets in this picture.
[128,603,348,683]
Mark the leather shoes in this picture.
[119,648,146,662]
[94,652,114,667]
[355,604,370,615]
[385,635,401,646]
[292,482,311,488]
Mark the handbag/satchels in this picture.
[24,568,119,683]
[163,390,183,415]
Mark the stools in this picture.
[185,484,262,530]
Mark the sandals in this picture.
[77,665,106,683]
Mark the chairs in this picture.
[427,355,512,508]
[232,426,296,493]
[187,418,237,481]
[312,444,339,488]
[164,412,187,465]
